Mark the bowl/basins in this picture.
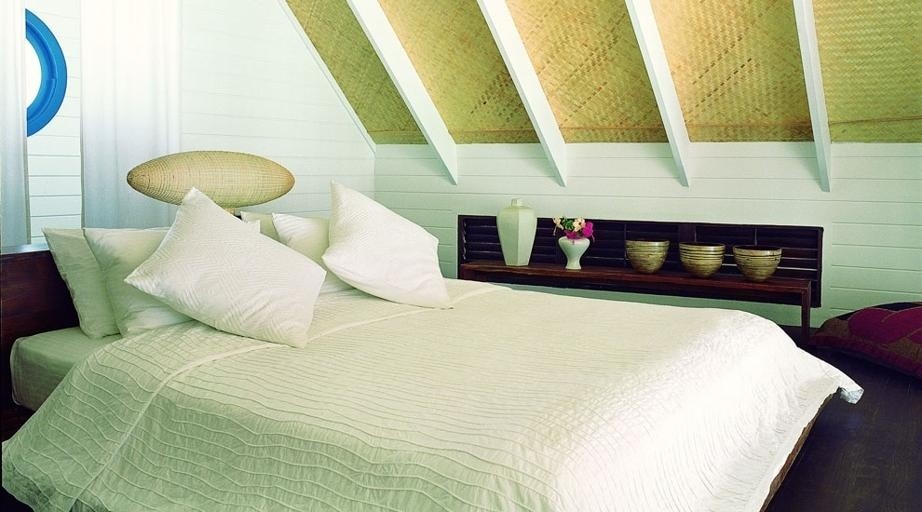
[733,245,782,282]
[679,242,726,278]
[624,238,670,274]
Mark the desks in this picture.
[459,257,816,344]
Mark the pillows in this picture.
[123,185,329,349]
[319,180,455,312]
[38,223,121,339]
[270,212,329,273]
[80,219,264,337]
[812,298,922,382]
[239,208,279,241]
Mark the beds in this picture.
[0,181,861,511]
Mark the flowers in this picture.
[550,214,598,241]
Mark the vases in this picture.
[557,235,590,270]
